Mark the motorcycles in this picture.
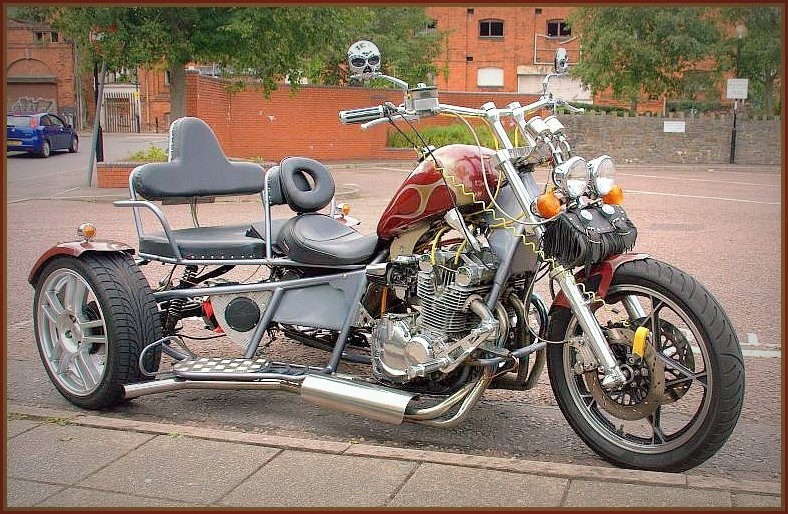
[28,40,746,472]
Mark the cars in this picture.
[7,111,79,157]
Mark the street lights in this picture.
[729,27,747,164]
